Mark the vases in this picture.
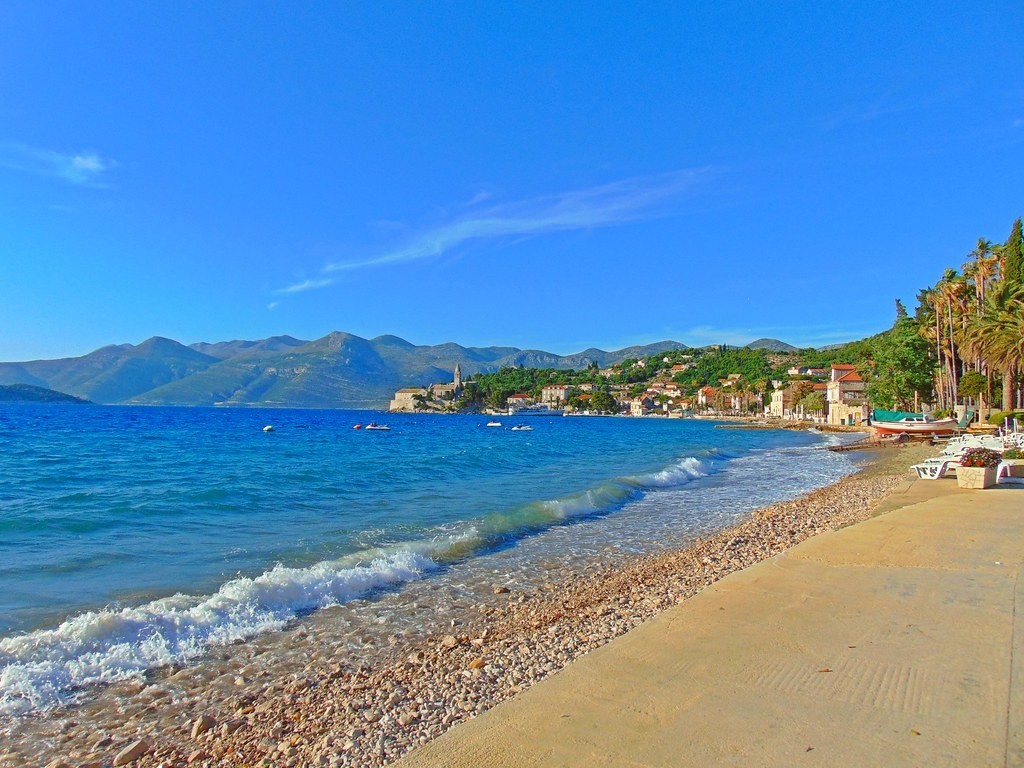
[954,464,998,489]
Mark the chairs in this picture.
[909,433,1024,485]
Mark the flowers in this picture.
[959,447,1003,470]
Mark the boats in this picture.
[871,418,957,434]
[366,422,391,429]
[487,421,501,426]
[512,424,534,431]
